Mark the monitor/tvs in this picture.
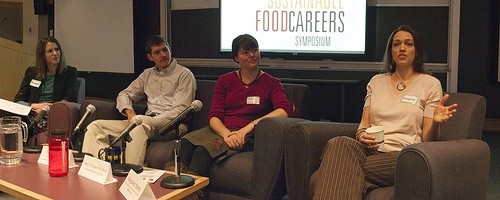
[219,0,367,59]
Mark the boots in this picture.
[163,161,205,200]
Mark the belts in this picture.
[148,112,161,117]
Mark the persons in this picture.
[13,34,79,149]
[81,34,199,166]
[308,24,459,200]
[166,34,295,200]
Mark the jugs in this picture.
[0,116,28,165]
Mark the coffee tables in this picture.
[0,149,209,200]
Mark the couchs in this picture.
[80,79,312,200]
[283,92,490,200]
[26,77,86,146]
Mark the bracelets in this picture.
[252,121,257,129]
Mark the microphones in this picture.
[109,116,142,147]
[28,105,50,128]
[72,104,96,136]
[158,100,203,136]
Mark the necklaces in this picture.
[394,67,415,91]
[239,67,261,86]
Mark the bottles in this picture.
[48,131,69,177]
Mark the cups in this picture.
[97,145,122,165]
[365,126,385,146]
